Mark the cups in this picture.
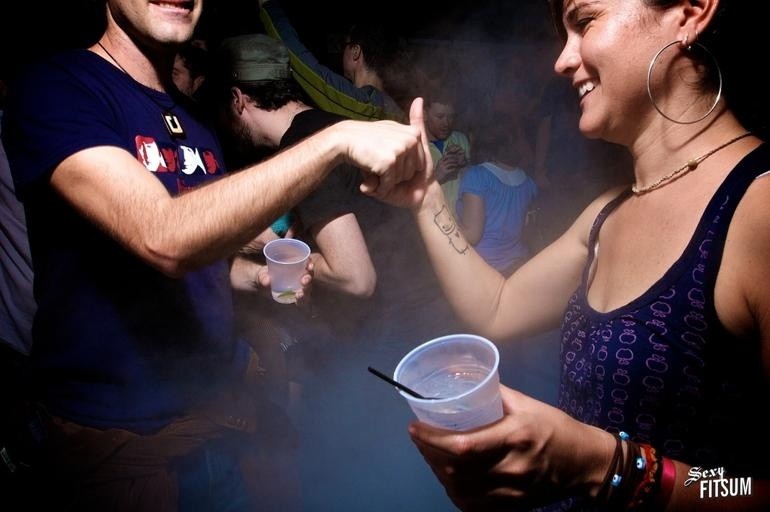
[264,238,311,305]
[392,332,506,470]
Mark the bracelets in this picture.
[590,427,676,511]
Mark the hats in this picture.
[236,32,294,81]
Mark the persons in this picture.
[169,41,208,99]
[4,1,426,512]
[1,140,37,357]
[257,1,411,126]
[360,1,769,512]
[224,35,465,375]
[422,92,470,223]
[455,110,539,279]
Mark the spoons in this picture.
[366,365,450,405]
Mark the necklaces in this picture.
[630,128,755,196]
[95,40,185,137]
